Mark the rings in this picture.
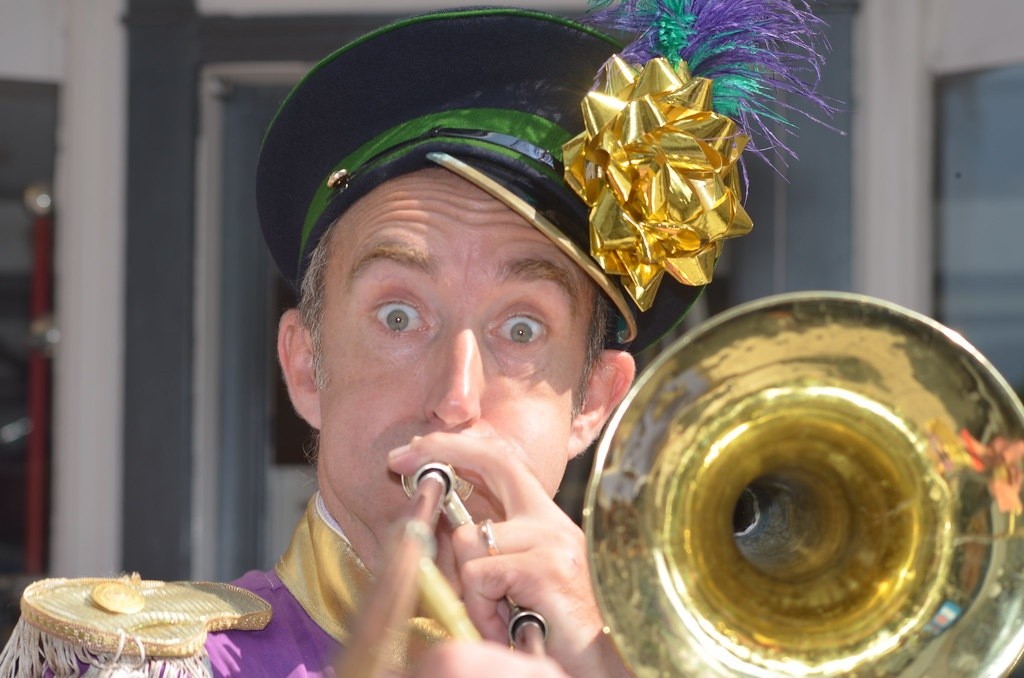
[480,518,498,555]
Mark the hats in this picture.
[254,0,851,357]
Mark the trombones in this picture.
[325,292,1023,678]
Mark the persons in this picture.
[0,8,752,678]
